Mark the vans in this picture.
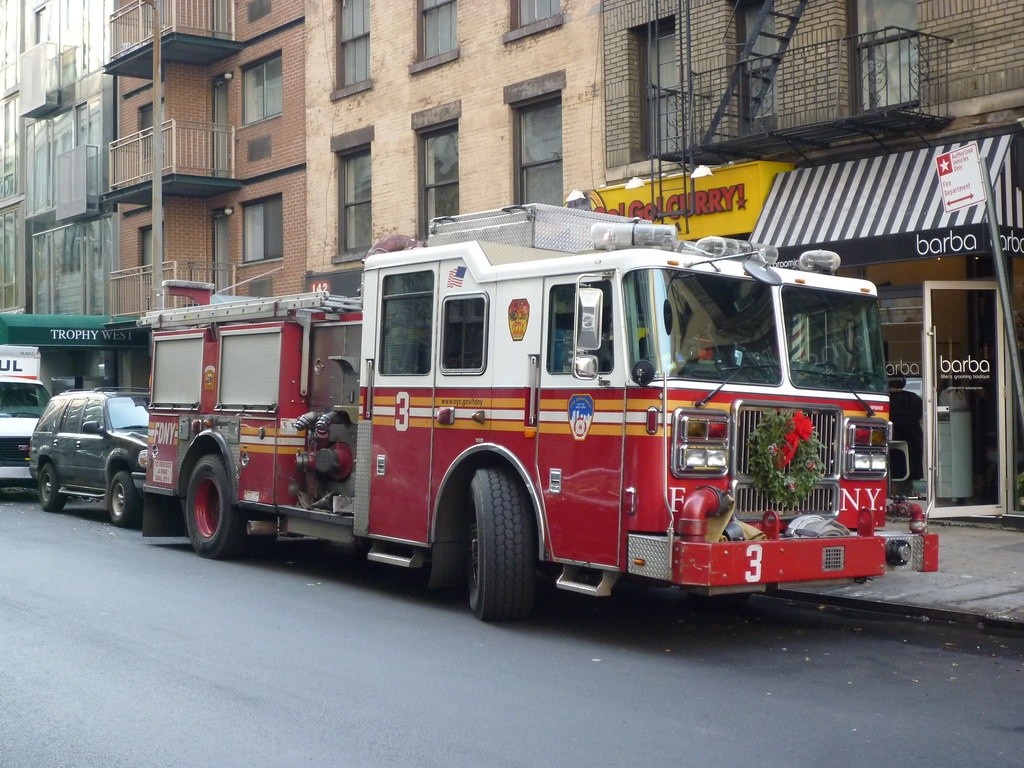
[0,378,53,490]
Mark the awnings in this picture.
[746,134,1024,271]
[0,313,150,348]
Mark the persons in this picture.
[887,370,923,499]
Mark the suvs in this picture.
[28,387,150,528]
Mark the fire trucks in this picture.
[140,200,940,624]
